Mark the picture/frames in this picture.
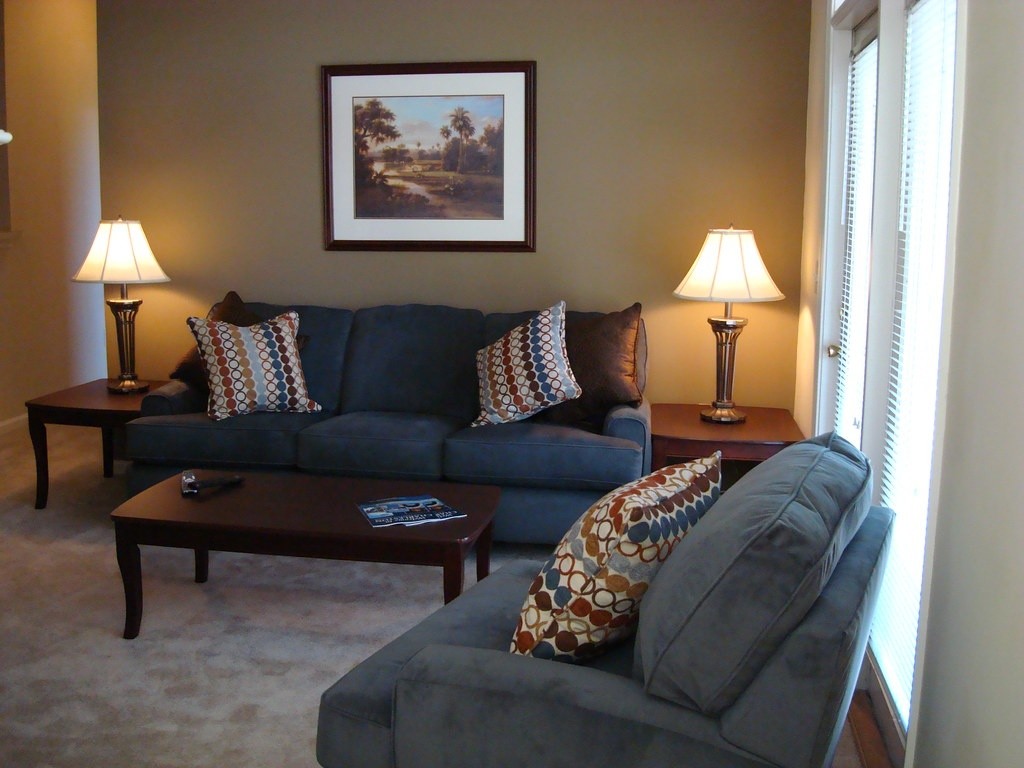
[321,60,537,254]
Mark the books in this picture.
[355,494,467,529]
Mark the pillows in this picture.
[169,290,311,399]
[633,431,873,717]
[543,303,643,426]
[509,449,724,664]
[185,309,322,421]
[472,299,583,426]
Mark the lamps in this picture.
[671,221,786,424]
[69,212,173,396]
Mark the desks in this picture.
[25,378,173,512]
[649,401,807,495]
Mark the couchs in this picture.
[316,506,895,768]
[123,301,652,545]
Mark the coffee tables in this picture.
[110,467,503,640]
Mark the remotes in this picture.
[187,475,246,488]
[182,470,198,494]
